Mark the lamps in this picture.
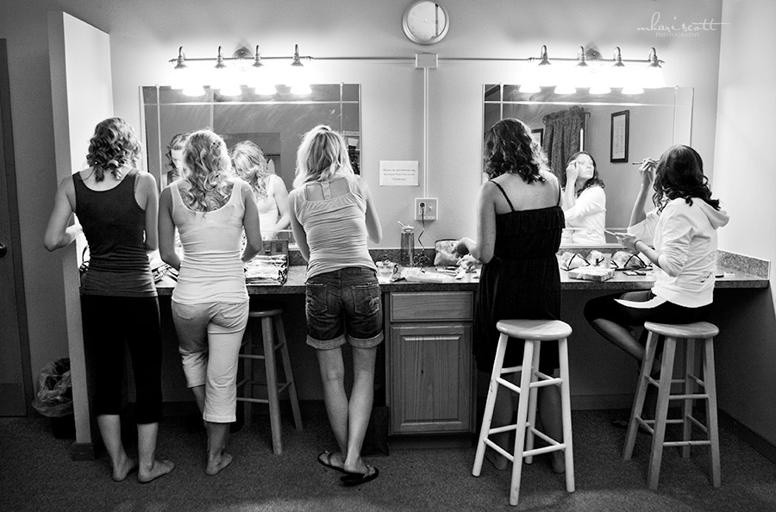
[170,43,663,70]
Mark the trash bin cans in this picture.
[31,357,76,439]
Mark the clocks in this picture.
[400,0,452,47]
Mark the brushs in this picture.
[631,157,662,166]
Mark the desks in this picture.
[94,257,475,444]
[475,261,770,290]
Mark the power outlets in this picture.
[415,197,438,221]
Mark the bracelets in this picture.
[633,239,642,250]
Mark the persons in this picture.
[447,116,570,475]
[283,124,384,490]
[155,132,196,191]
[580,142,731,439]
[227,139,292,243]
[154,125,263,479]
[41,117,176,485]
[562,149,609,247]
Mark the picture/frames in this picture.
[609,109,631,162]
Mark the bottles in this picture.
[400,225,415,268]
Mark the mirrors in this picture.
[138,80,361,252]
[479,82,695,250]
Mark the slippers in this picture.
[339,466,379,487]
[317,450,345,472]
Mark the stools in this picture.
[621,316,726,493]
[472,318,576,504]
[235,307,302,458]
